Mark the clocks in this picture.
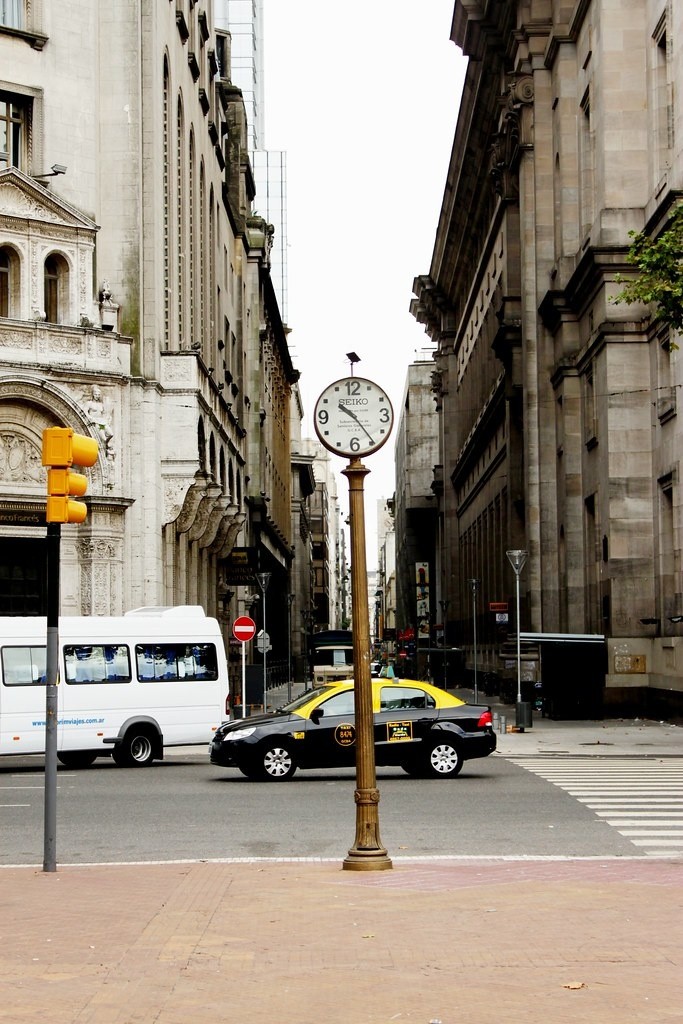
[314,376,393,456]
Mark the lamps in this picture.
[640,618,660,624]
[227,403,232,406]
[242,428,246,434]
[33,164,68,177]
[218,383,224,389]
[209,368,215,372]
[667,616,683,623]
[260,491,266,496]
[191,342,201,349]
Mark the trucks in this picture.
[310,630,355,689]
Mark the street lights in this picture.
[467,577,482,705]
[506,549,528,732]
[256,572,273,712]
[439,600,450,693]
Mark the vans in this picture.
[0,605,233,768]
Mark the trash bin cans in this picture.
[232,704,252,720]
[515,701,532,728]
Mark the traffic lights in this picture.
[42,425,99,524]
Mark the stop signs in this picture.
[233,616,256,642]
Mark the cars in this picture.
[208,676,497,782]
